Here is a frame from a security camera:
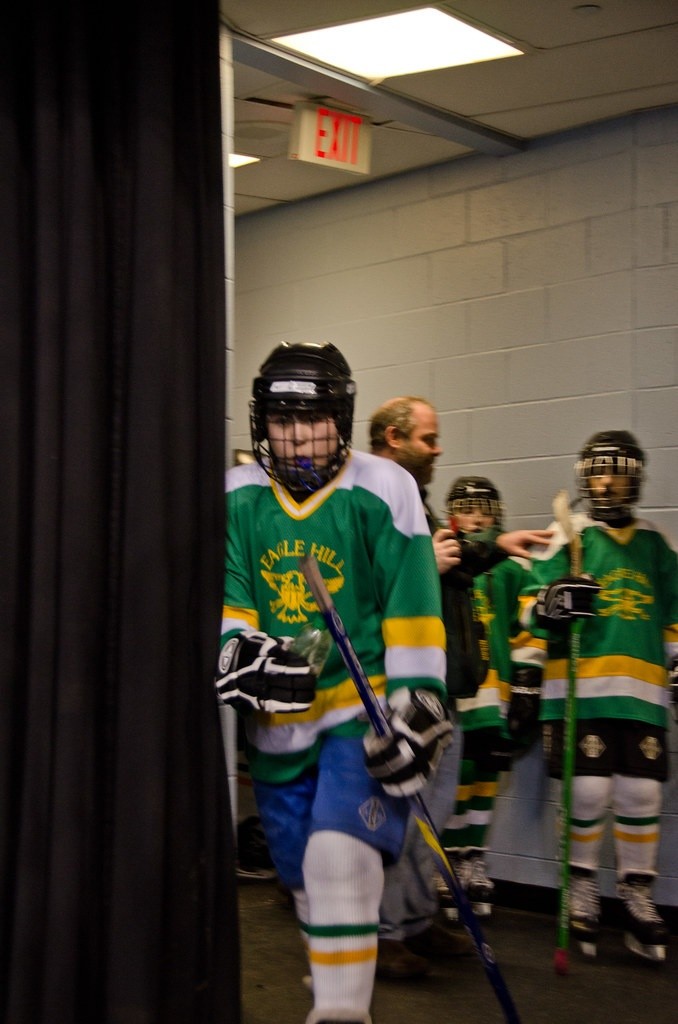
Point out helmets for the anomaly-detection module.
[446,475,504,557]
[248,340,356,490]
[570,430,644,519]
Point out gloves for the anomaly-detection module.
[507,665,543,760]
[361,686,455,798]
[537,577,601,626]
[666,653,678,722]
[213,629,317,714]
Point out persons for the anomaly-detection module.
[519,429,678,965]
[365,395,555,987]
[214,340,453,1024]
[433,473,549,923]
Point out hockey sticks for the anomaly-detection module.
[552,491,581,975]
[297,553,522,1024]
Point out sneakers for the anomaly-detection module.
[434,851,498,908]
[568,867,601,941]
[376,938,429,978]
[403,923,474,958]
[236,814,278,878]
[614,874,669,945]
